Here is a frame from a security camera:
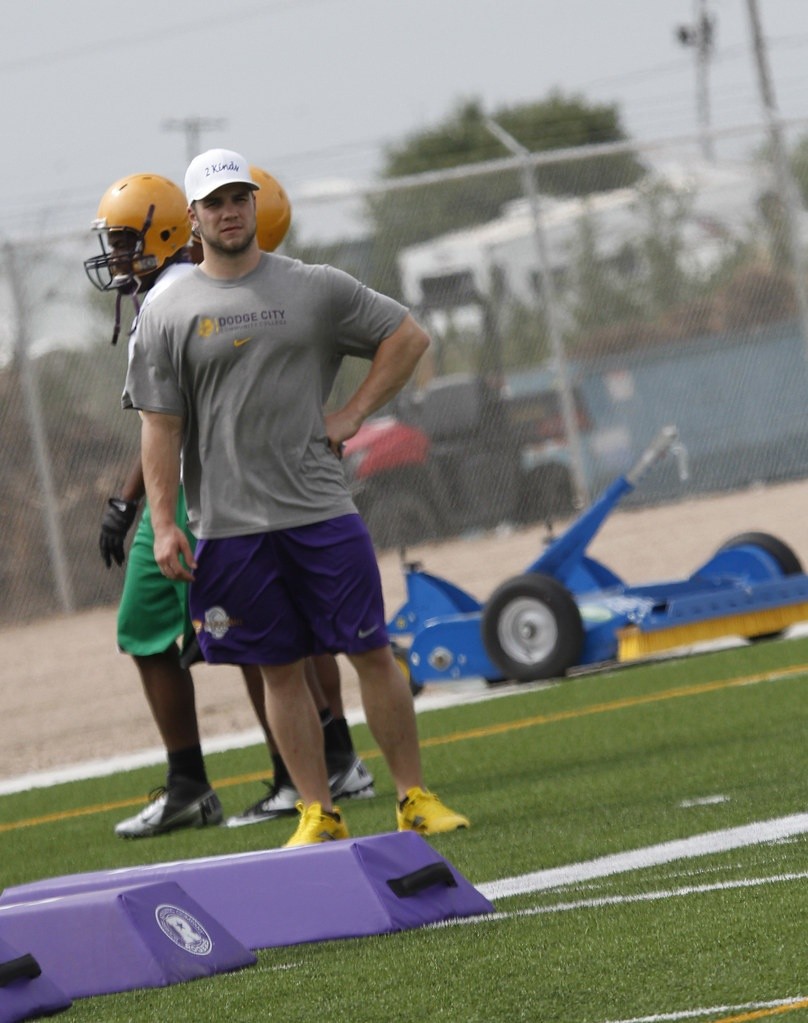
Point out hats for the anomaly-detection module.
[185,149,260,209]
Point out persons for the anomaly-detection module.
[122,148,470,847]
[84,166,377,838]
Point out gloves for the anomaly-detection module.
[98,499,137,569]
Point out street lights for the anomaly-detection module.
[450,101,591,514]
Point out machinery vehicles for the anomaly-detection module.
[341,274,593,552]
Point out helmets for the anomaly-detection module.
[248,166,289,254]
[91,175,192,273]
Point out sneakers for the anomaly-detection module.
[115,774,223,839]
[327,756,376,804]
[395,789,469,833]
[223,782,304,827]
[283,799,349,846]
